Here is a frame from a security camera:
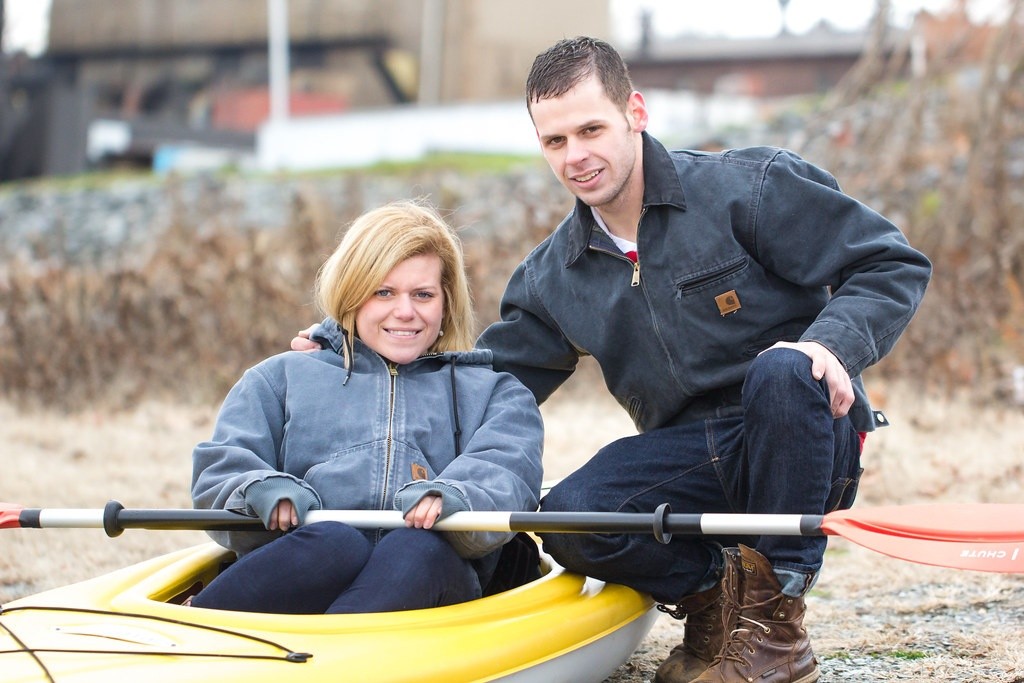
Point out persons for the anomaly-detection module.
[178,198,545,613]
[290,32,932,683]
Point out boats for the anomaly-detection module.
[1,526,667,683]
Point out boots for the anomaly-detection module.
[689,543,822,683]
[650,581,722,683]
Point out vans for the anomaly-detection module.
[0,30,423,187]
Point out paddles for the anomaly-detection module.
[0,503,1023,576]
